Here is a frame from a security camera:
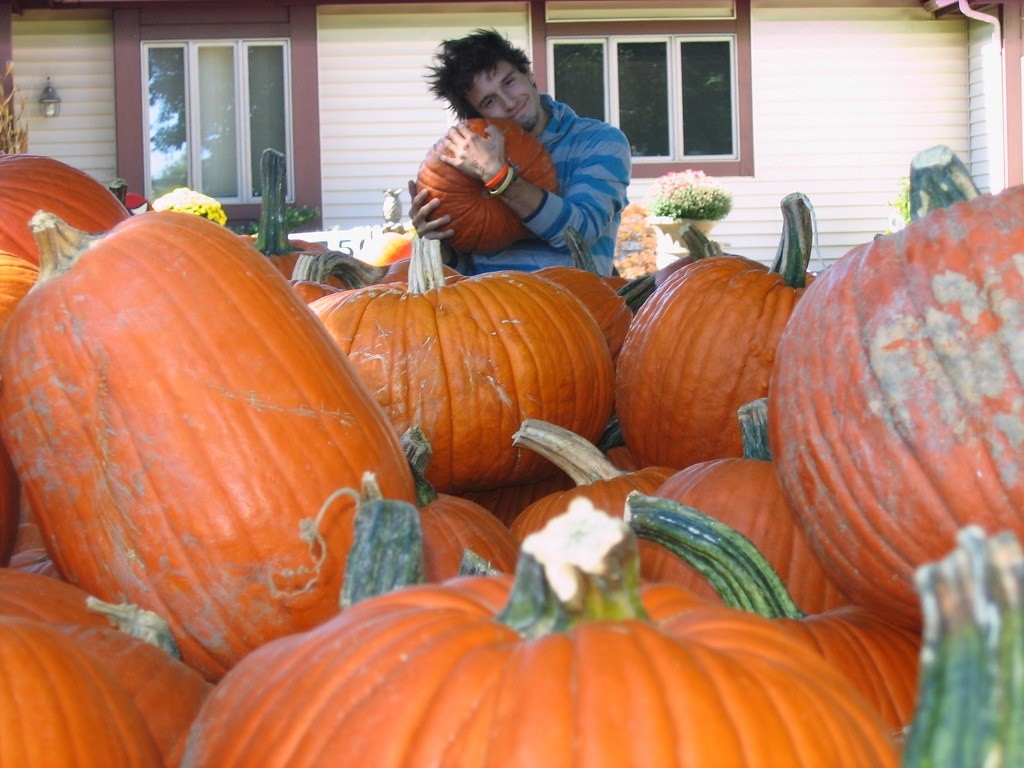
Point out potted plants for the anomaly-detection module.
[653,217,717,261]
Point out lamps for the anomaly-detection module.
[37,74,63,119]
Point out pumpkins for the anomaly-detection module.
[1,120,1024,768]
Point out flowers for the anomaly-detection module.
[151,188,227,226]
[649,168,733,220]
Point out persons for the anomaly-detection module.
[408,27,631,279]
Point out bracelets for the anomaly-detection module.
[485,161,514,197]
